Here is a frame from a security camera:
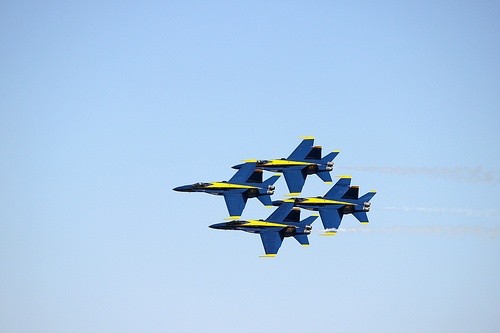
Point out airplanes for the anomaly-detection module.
[171,159,282,220]
[272,175,376,235]
[209,199,319,256]
[230,137,340,196]
